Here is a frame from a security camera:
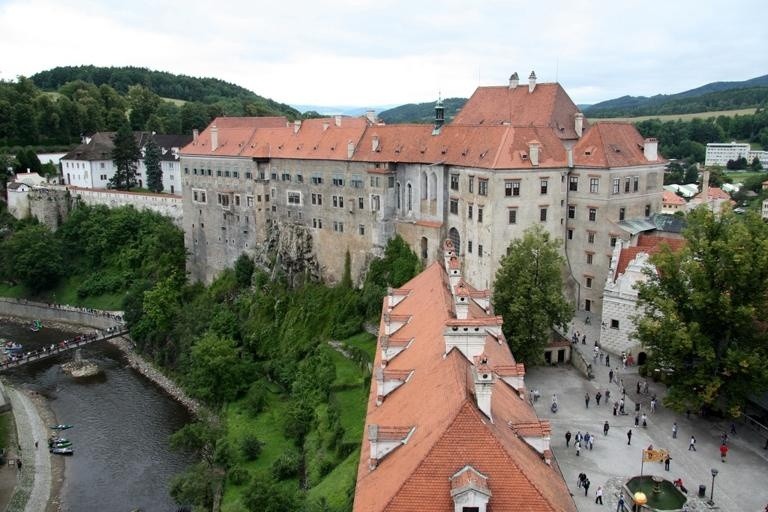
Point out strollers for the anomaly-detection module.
[551,400,558,412]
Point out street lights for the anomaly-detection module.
[654,365,679,407]
[709,468,718,506]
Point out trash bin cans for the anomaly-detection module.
[635,402,640,411]
[595,341,597,346]
[699,485,705,497]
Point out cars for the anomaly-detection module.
[733,208,745,213]
[742,199,751,206]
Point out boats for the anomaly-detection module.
[30,326,40,332]
[0,338,6,347]
[33,319,42,329]
[48,424,73,430]
[5,342,25,350]
[49,435,74,455]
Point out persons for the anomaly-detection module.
[595,485,604,505]
[664,453,672,471]
[672,422,678,439]
[17,458,22,471]
[631,502,641,512]
[570,317,660,444]
[530,386,539,401]
[730,424,736,434]
[647,444,654,458]
[616,491,625,512]
[582,478,590,496]
[551,393,558,407]
[674,477,687,494]
[688,436,696,451]
[5,298,122,361]
[719,431,728,463]
[565,431,595,456]
[578,470,586,488]
[686,409,691,419]
